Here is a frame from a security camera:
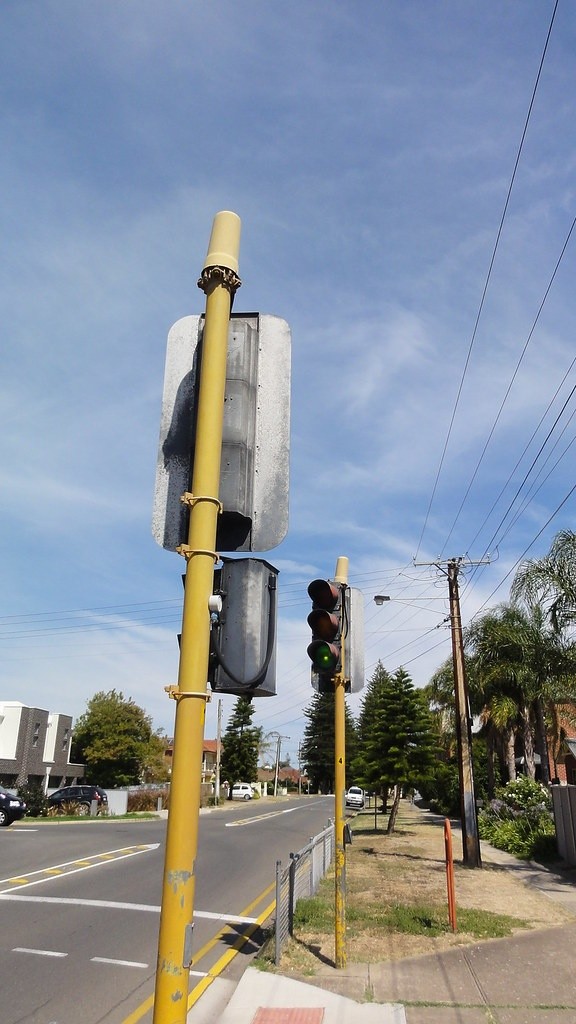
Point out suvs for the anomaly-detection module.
[47,785,110,809]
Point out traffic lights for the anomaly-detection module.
[307,578,351,679]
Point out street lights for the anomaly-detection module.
[372,593,483,873]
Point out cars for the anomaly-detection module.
[226,782,255,800]
[0,785,28,827]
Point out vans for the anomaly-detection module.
[346,786,364,807]
